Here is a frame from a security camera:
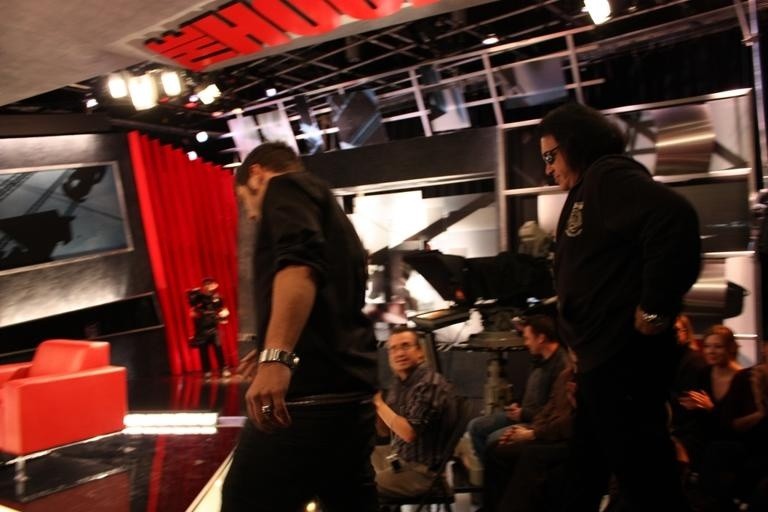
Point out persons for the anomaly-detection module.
[482,341,590,512]
[187,278,231,378]
[374,328,460,512]
[668,315,703,421]
[468,318,578,467]
[220,142,381,512]
[539,104,680,512]
[678,324,758,438]
[721,365,768,512]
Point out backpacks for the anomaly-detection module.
[642,178,702,298]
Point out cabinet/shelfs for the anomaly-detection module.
[493,88,764,370]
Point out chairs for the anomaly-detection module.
[379,416,468,511]
[0,338,128,503]
[436,350,447,378]
[501,346,546,407]
[374,330,438,440]
[448,347,503,427]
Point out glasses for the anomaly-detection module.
[389,343,416,356]
[541,142,563,167]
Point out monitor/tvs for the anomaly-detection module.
[0,159,135,276]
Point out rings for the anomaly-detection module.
[261,404,273,416]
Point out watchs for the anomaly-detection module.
[257,349,302,377]
[642,312,660,325]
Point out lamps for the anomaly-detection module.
[87,99,98,110]
[161,69,184,96]
[128,74,157,111]
[580,0,615,24]
[196,131,210,142]
[196,90,213,106]
[206,83,221,98]
[189,93,197,102]
[265,87,276,95]
[108,72,127,100]
[482,34,498,48]
